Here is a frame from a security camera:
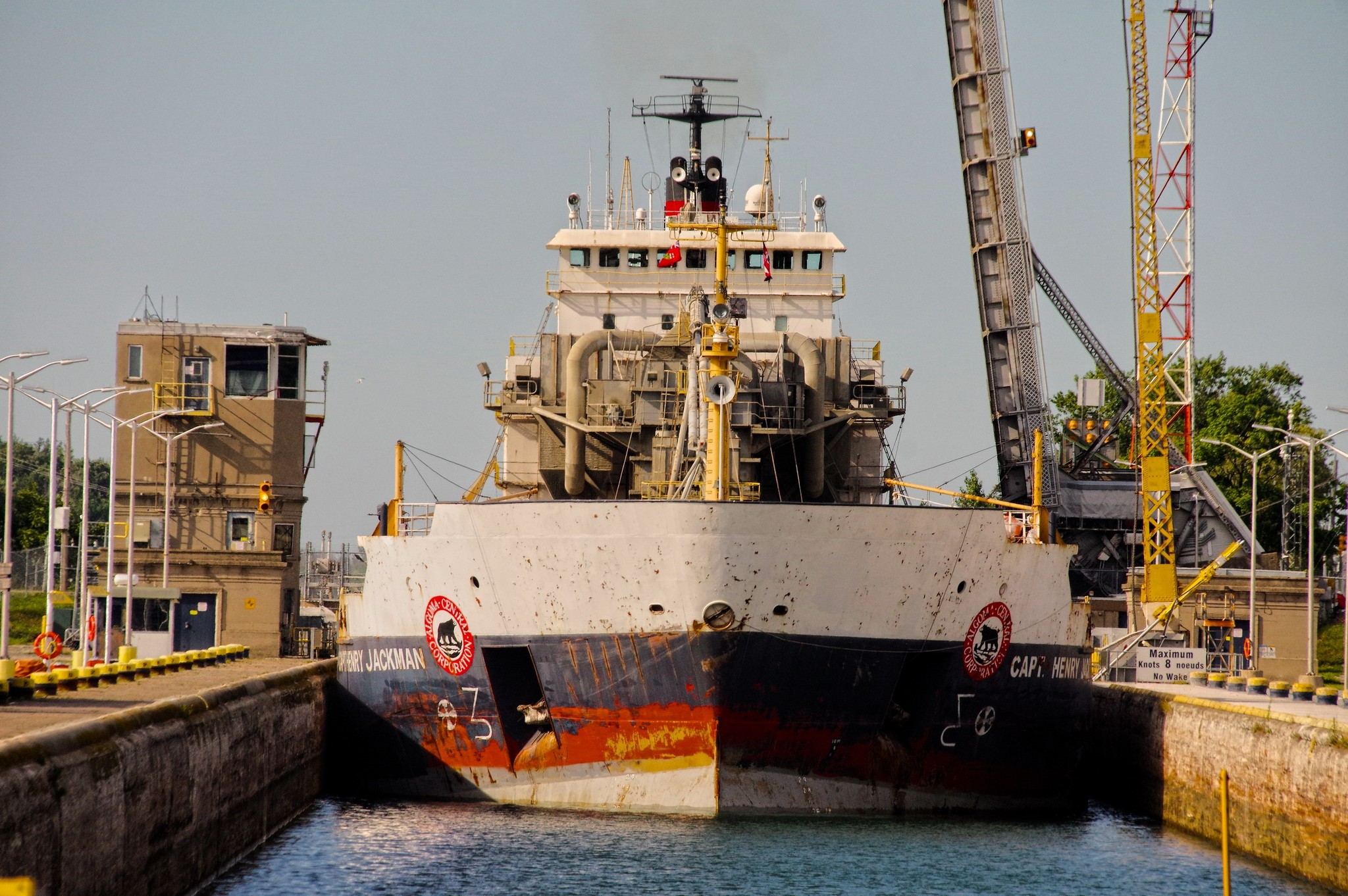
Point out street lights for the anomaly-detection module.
[58,407,178,664]
[0,383,127,673]
[116,420,224,589]
[1199,438,1304,668]
[1251,422,1347,677]
[0,358,90,659]
[21,386,154,666]
[88,408,194,645]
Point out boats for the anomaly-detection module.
[328,76,1095,825]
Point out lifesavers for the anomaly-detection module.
[33,631,63,660]
[1244,638,1251,660]
[88,615,95,641]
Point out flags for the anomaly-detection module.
[762,242,773,282]
[656,239,682,267]
